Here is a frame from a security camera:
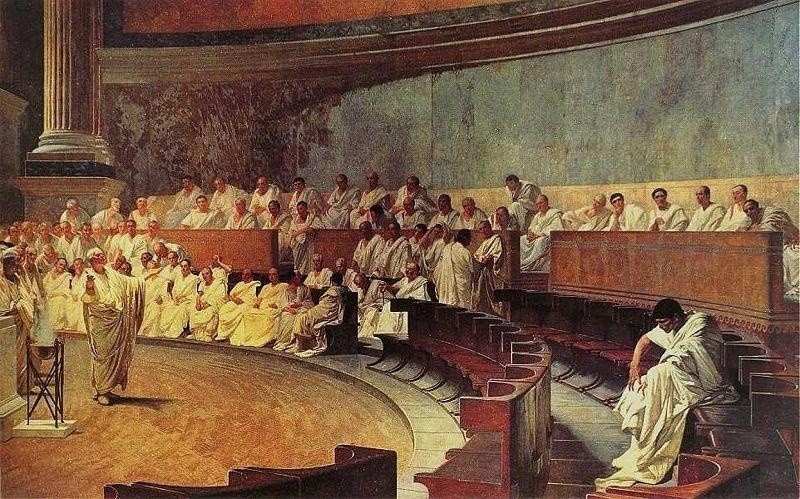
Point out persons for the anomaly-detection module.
[0,247,42,395]
[81,247,148,406]
[1,171,798,373]
[618,297,722,461]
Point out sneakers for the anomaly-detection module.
[97,393,119,405]
